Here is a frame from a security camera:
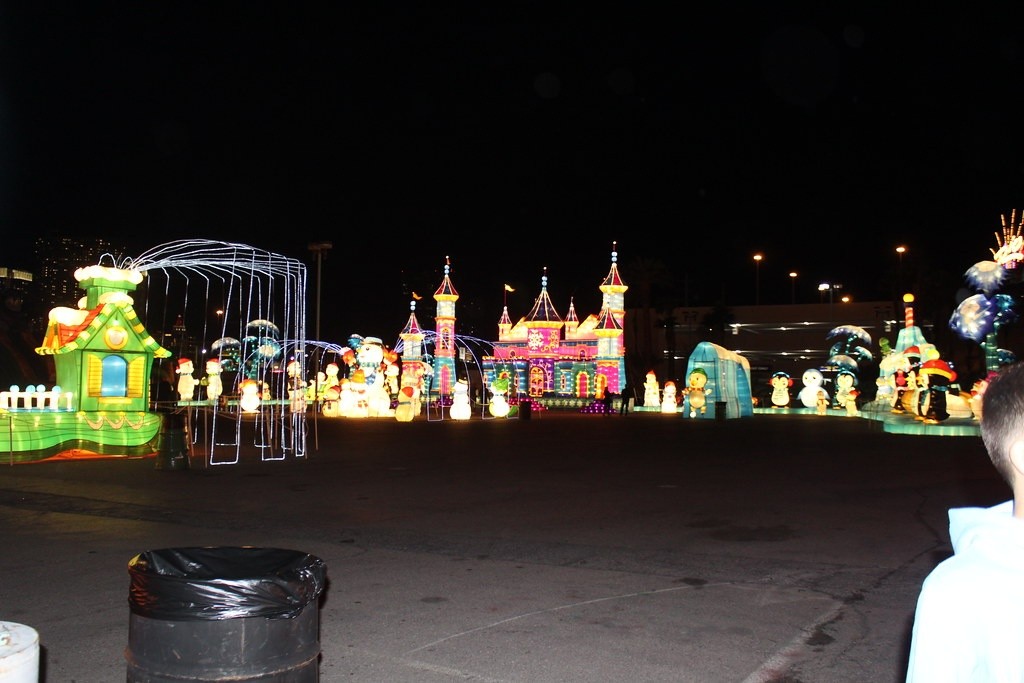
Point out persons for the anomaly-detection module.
[903,363,1024,682]
[618,384,630,416]
[603,387,612,418]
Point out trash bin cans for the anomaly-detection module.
[123,546,330,683]
